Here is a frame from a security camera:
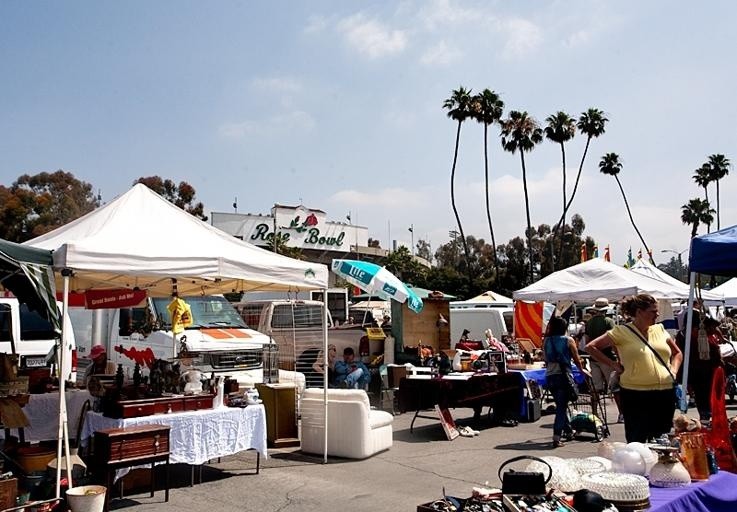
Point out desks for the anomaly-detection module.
[80,404,268,502]
[506,357,592,423]
[646,468,725,511]
[0,389,97,448]
[397,367,528,434]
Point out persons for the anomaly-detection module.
[484,328,509,351]
[312,344,336,388]
[721,309,737,339]
[675,310,725,420]
[380,315,392,331]
[343,316,355,325]
[676,297,700,330]
[583,294,682,443]
[585,297,624,424]
[334,347,372,389]
[725,374,737,401]
[543,316,592,447]
[83,345,117,386]
[677,299,689,337]
[458,330,470,343]
[570,334,579,349]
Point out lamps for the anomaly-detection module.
[581,472,650,509]
[649,447,691,487]
[1,400,33,464]
[436,312,449,349]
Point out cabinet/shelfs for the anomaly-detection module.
[379,382,399,416]
[254,383,301,448]
[93,424,172,512]
[103,391,214,418]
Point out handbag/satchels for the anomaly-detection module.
[547,336,579,401]
[621,324,690,412]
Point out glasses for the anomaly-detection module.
[328,347,336,352]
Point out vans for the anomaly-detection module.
[0,299,77,383]
[450,307,513,348]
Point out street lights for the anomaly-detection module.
[660,250,689,292]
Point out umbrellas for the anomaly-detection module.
[330,260,423,327]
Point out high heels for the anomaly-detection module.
[567,433,578,440]
[553,441,566,447]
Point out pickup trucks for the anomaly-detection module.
[235,300,388,368]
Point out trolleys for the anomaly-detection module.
[560,368,610,442]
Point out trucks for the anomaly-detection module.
[64,294,278,380]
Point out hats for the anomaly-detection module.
[88,345,106,358]
[344,347,356,353]
[586,298,616,311]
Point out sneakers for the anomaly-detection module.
[617,414,624,423]
[459,425,480,435]
[456,427,474,437]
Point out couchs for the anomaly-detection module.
[300,388,394,459]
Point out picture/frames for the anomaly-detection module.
[488,352,504,365]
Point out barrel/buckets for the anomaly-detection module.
[66,484,108,512]
